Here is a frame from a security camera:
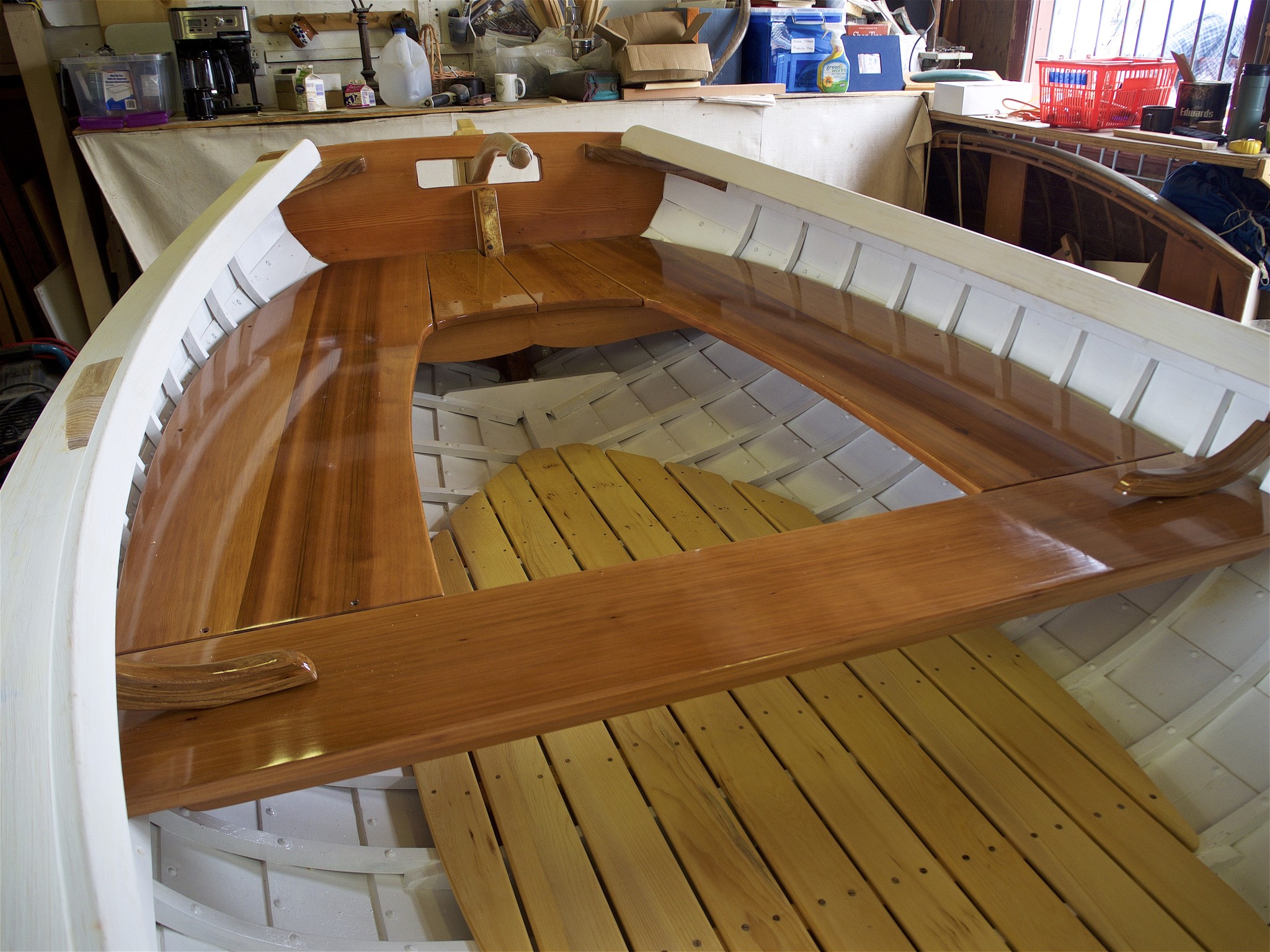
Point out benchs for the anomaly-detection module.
[115,234,1270,812]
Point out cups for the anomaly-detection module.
[445,13,468,44]
[452,77,485,102]
[183,87,218,120]
[1140,105,1177,134]
[495,73,526,102]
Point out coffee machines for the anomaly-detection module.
[168,5,263,121]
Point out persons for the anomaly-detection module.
[1157,10,1250,117]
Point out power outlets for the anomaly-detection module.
[249,43,267,75]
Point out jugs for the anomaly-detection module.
[185,47,239,94]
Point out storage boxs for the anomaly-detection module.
[934,82,1033,116]
[61,52,172,128]
[594,0,845,100]
[274,73,344,111]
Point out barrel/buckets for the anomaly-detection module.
[1174,79,1231,132]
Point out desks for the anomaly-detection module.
[73,88,946,307]
[926,110,1270,310]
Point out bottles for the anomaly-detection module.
[1227,63,1270,142]
[378,28,432,107]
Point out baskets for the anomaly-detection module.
[1035,56,1179,132]
